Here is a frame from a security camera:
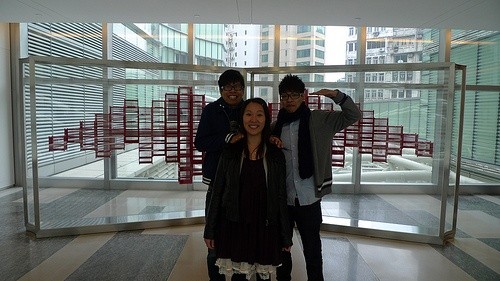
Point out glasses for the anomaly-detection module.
[280,91,303,101]
[221,83,243,91]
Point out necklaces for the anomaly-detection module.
[248,144,258,154]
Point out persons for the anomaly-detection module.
[204,98,293,281]
[270,74,361,281]
[194,70,284,281]
